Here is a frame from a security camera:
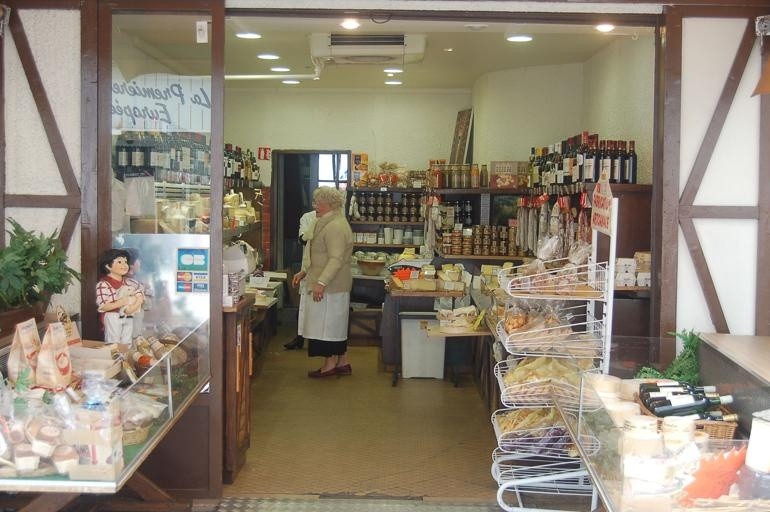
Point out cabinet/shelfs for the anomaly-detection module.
[339,178,655,423]
[214,287,257,486]
[483,190,621,512]
[115,166,278,365]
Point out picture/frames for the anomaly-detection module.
[446,105,476,167]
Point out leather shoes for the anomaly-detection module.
[335,363,352,375]
[308,367,336,377]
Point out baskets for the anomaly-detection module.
[632,392,739,452]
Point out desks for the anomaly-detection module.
[0,303,217,512]
[543,332,769,512]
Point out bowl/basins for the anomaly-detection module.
[351,225,424,246]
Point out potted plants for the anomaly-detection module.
[0,211,90,340]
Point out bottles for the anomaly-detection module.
[508,226,517,242]
[440,200,473,227]
[526,130,639,186]
[112,127,261,188]
[356,191,425,222]
[122,331,184,367]
[427,159,490,189]
[518,245,524,256]
[637,374,743,428]
[508,242,517,257]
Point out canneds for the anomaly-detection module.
[359,192,425,222]
[442,200,517,256]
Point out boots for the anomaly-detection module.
[283,336,304,349]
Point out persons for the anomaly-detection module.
[96,248,143,349]
[282,210,320,350]
[293,186,353,377]
